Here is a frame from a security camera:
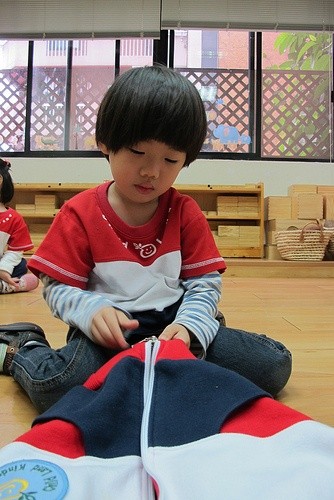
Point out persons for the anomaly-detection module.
[0,64,293,415]
[0,158,39,294]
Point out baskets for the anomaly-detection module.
[316,219,334,252]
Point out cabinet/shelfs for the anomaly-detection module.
[11,182,266,258]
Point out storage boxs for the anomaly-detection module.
[16,195,69,256]
[203,195,259,217]
[265,184,334,259]
[211,225,260,247]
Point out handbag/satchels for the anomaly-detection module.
[275,222,333,261]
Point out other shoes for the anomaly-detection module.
[215,312,226,326]
[0,321,46,376]
